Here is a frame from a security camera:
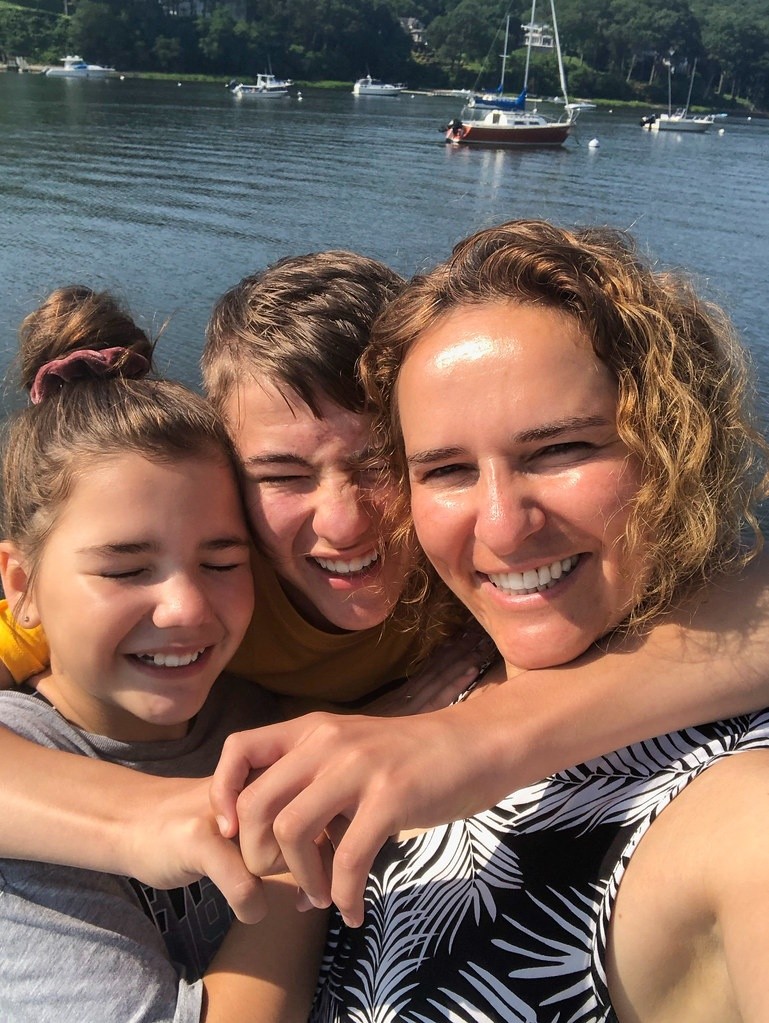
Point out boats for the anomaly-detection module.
[564,103,600,111]
[224,55,295,98]
[351,75,407,96]
[40,54,116,78]
[639,47,714,132]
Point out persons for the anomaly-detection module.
[0,282,491,1023]
[305,217,768,1023]
[1,246,769,930]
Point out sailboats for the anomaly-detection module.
[439,0,576,147]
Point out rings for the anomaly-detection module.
[403,694,412,702]
